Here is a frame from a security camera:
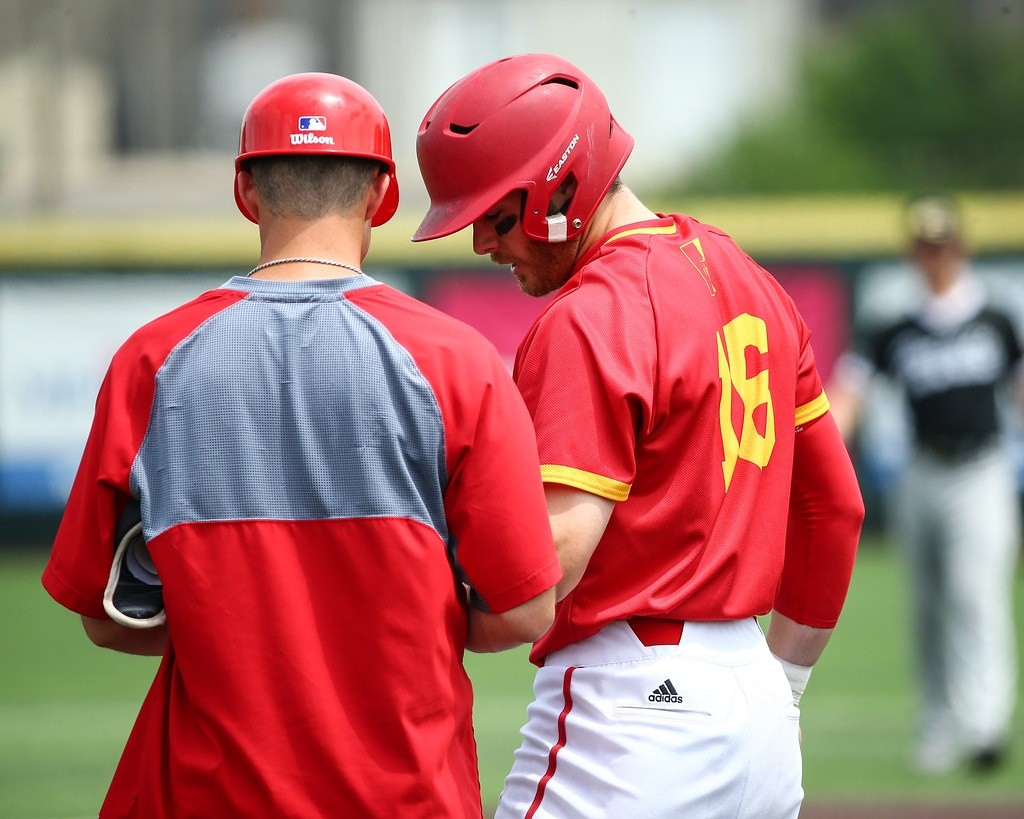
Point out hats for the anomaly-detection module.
[902,193,964,245]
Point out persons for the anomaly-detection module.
[40,73,564,819]
[408,53,865,819]
[818,190,1024,780]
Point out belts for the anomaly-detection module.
[625,615,684,646]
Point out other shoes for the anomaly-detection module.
[969,742,1012,775]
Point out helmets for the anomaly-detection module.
[234,73,400,228]
[410,53,635,242]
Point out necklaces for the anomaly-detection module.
[246,258,363,277]
